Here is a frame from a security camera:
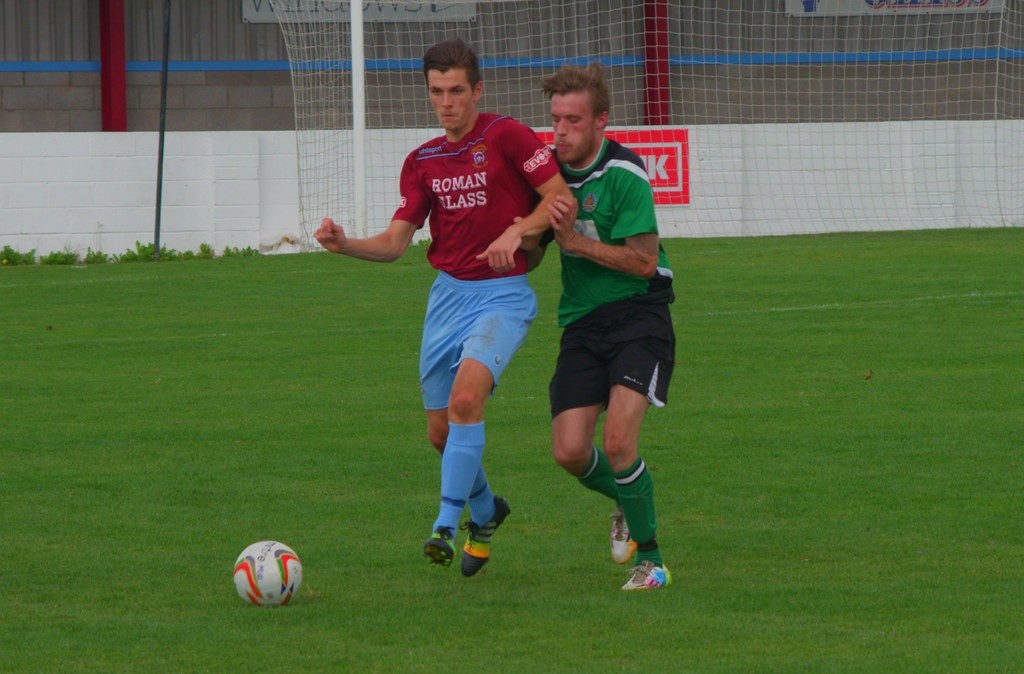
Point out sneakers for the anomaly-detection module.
[608,506,637,564]
[620,560,672,590]
[459,497,511,577]
[423,525,457,567]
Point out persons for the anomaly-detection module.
[315,38,571,578]
[512,66,677,592]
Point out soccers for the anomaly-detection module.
[231,539,303,604]
